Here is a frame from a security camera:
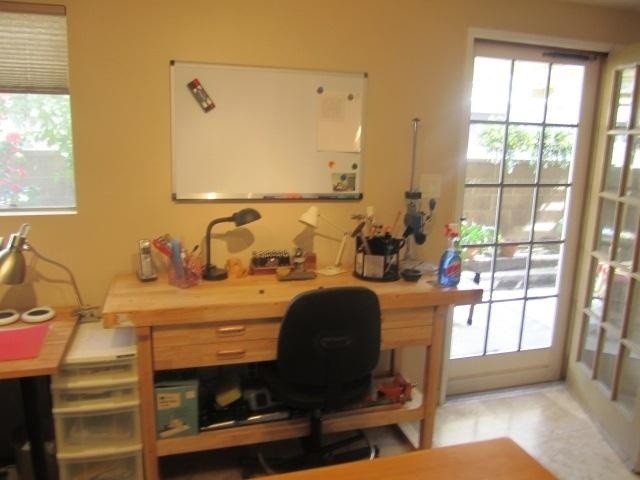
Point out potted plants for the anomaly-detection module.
[460,221,487,261]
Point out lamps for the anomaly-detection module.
[0,234,95,321]
[299,206,350,276]
[203,209,262,280]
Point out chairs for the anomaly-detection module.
[256,288,380,476]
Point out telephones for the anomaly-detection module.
[138,240,157,280]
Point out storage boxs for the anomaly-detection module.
[156,376,199,439]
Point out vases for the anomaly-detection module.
[500,240,519,256]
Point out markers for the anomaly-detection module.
[263,193,363,201]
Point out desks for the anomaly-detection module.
[254,438,561,480]
[0,306,81,479]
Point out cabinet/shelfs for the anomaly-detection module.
[51,309,144,480]
[104,271,482,480]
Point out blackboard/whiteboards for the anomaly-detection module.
[169,60,368,201]
